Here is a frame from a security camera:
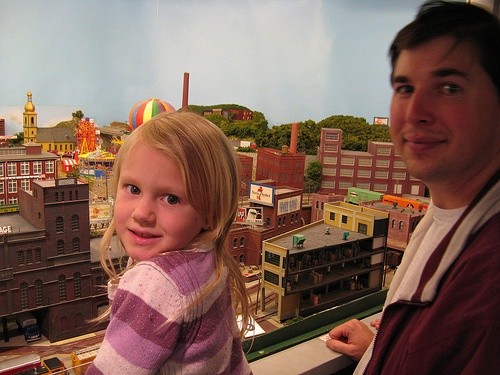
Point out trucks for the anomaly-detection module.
[0,355,66,375]
[71,342,103,375]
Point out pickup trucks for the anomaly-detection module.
[17,315,41,342]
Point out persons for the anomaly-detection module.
[85,111,253,375]
[326,0,500,375]
